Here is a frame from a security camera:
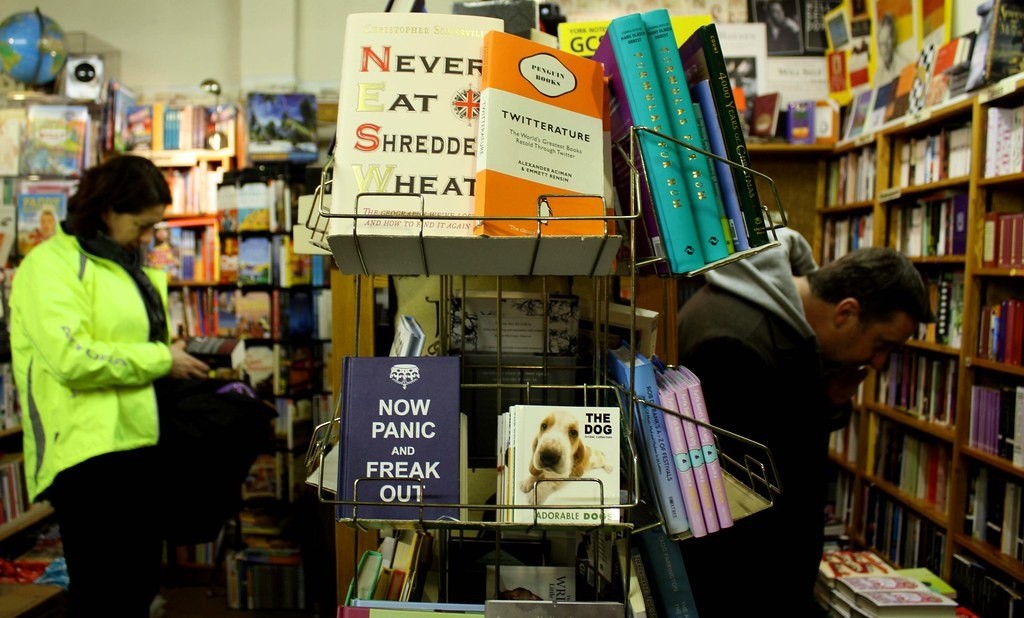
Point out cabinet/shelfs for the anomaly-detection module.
[1,92,336,617]
[812,72,1023,618]
[305,118,788,618]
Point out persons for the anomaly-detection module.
[652,226,940,618]
[8,154,215,618]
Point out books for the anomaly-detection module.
[326,13,772,618]
[689,0,1024,617]
[0,88,329,616]
[169,333,247,373]
